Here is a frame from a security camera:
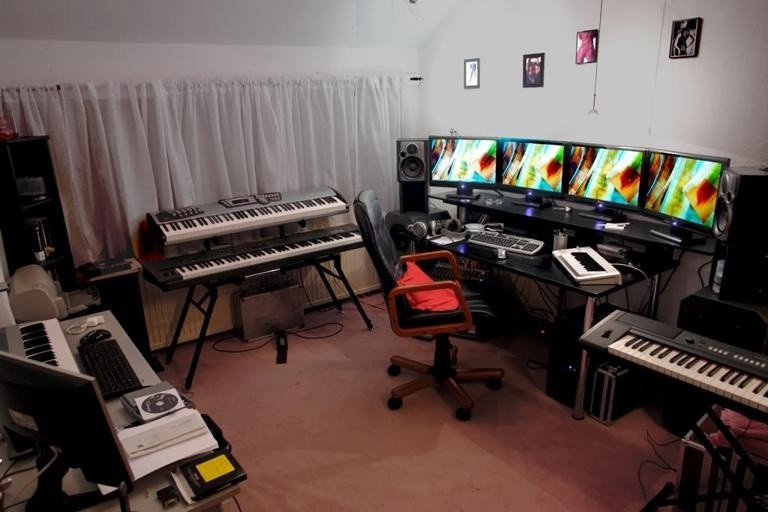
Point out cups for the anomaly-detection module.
[553,235,568,252]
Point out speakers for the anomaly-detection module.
[709,166,768,300]
[396,138,429,215]
[384,211,428,257]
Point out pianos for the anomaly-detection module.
[145,185,349,245]
[579,309,768,422]
[141,221,365,293]
[1,318,82,459]
[552,246,623,286]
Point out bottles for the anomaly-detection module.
[33,227,47,264]
[712,260,725,294]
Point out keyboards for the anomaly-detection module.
[467,228,544,256]
[75,339,143,402]
[429,266,492,282]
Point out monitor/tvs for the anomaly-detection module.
[566,141,647,222]
[0,348,135,512]
[500,137,567,208]
[642,147,730,244]
[428,137,503,198]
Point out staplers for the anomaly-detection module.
[484,223,504,232]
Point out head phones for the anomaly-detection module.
[408,220,443,238]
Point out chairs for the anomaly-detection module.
[353,189,505,421]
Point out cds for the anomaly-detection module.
[141,393,178,413]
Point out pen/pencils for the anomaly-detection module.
[553,228,567,237]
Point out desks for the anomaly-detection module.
[399,194,724,420]
[77,255,152,361]
[0,309,241,512]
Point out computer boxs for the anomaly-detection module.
[545,304,625,410]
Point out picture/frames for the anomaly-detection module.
[523,53,545,88]
[464,58,480,89]
[669,16,703,59]
[575,29,599,64]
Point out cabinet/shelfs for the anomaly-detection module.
[0,135,81,295]
[167,252,373,389]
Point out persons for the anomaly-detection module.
[469,63,476,81]
[673,26,695,56]
[527,58,539,83]
[576,30,595,63]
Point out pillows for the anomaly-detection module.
[397,262,461,312]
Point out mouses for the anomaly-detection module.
[76,328,111,343]
[495,248,508,260]
